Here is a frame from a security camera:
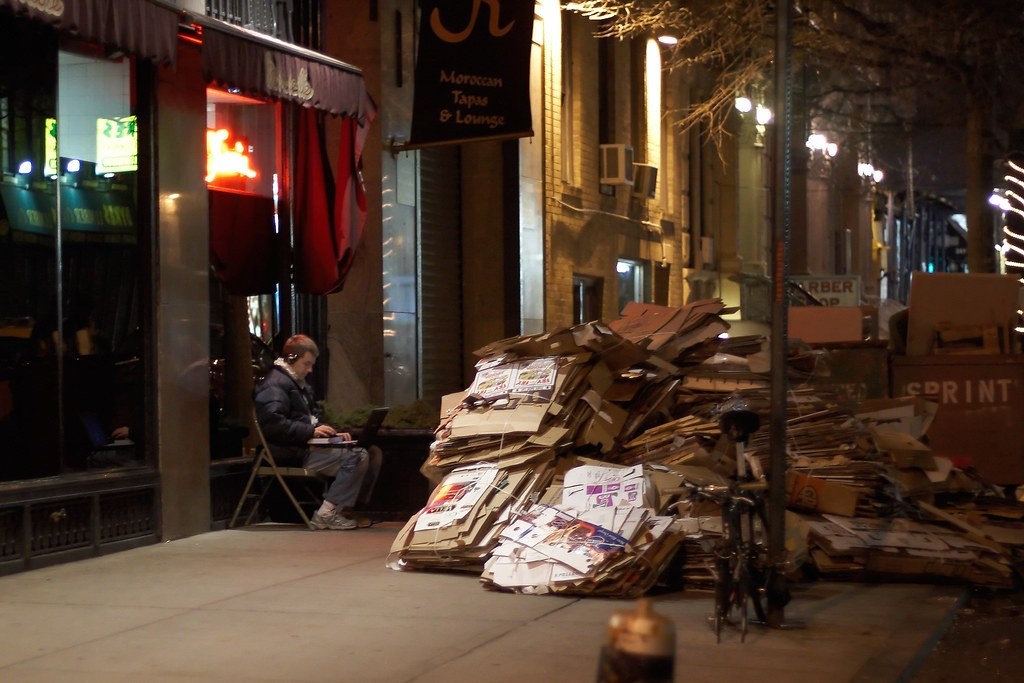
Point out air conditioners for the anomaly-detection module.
[632,162,658,200]
[597,144,634,186]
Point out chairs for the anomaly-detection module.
[229,408,330,532]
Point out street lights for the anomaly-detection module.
[20,157,83,471]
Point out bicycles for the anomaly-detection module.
[659,397,790,644]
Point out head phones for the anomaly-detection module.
[287,353,297,364]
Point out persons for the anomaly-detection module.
[86,421,144,470]
[252,335,382,529]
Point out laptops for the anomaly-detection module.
[307,406,391,448]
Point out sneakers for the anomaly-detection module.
[309,508,357,529]
[345,512,374,527]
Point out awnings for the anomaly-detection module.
[0,0,367,128]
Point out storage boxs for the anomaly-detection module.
[389,270,1024,599]
